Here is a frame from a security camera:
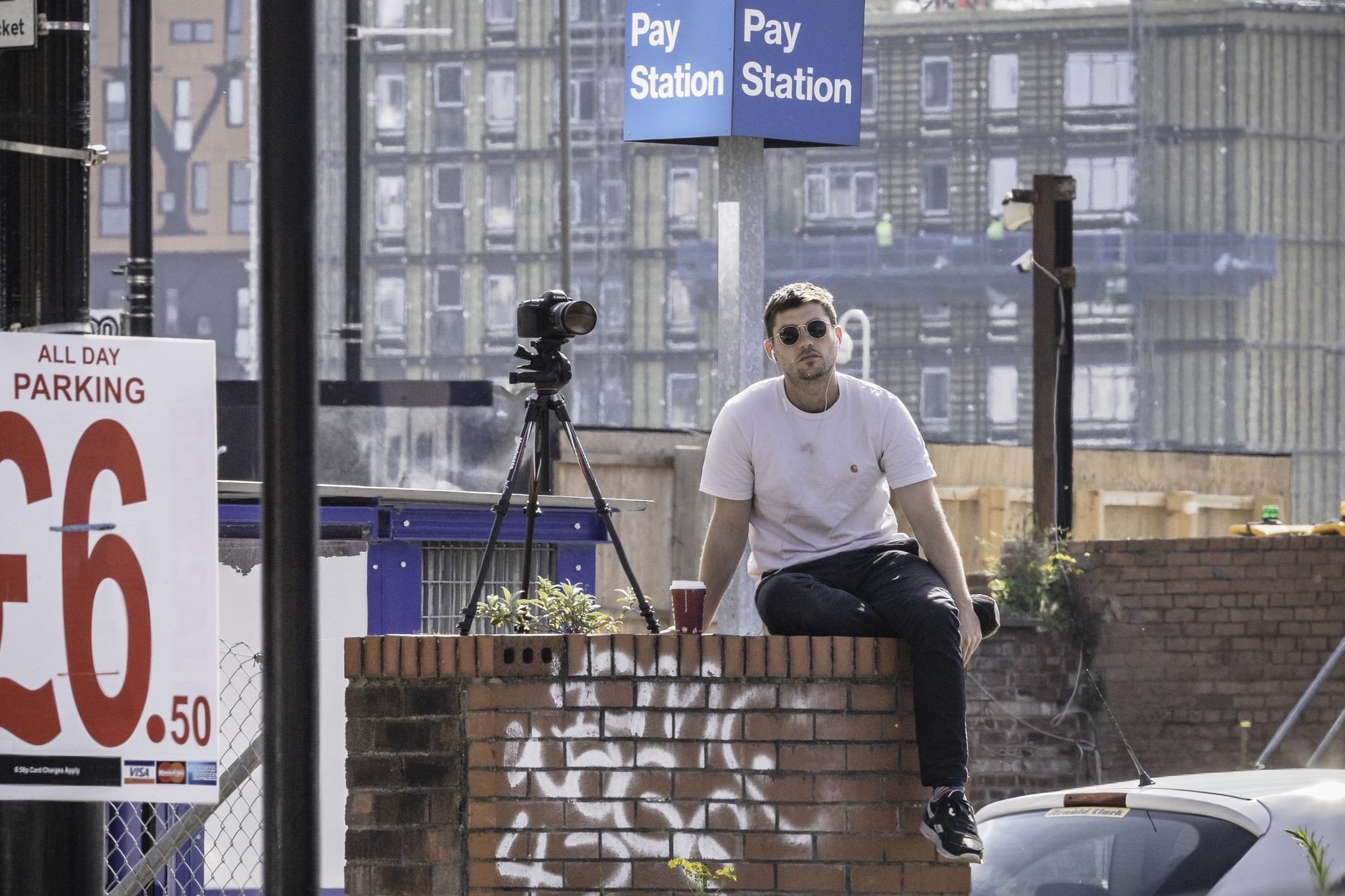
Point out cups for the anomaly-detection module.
[670,580,707,634]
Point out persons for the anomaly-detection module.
[659,281,1002,864]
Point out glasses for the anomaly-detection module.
[769,319,835,346]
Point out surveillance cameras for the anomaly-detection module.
[1011,247,1034,273]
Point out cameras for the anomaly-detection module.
[517,288,598,339]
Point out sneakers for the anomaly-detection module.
[971,593,1001,640]
[918,789,985,864]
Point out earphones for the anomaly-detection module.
[771,348,776,362]
[836,336,840,349]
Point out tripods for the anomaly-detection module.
[454,336,660,637]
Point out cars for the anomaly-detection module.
[968,767,1345,895]
